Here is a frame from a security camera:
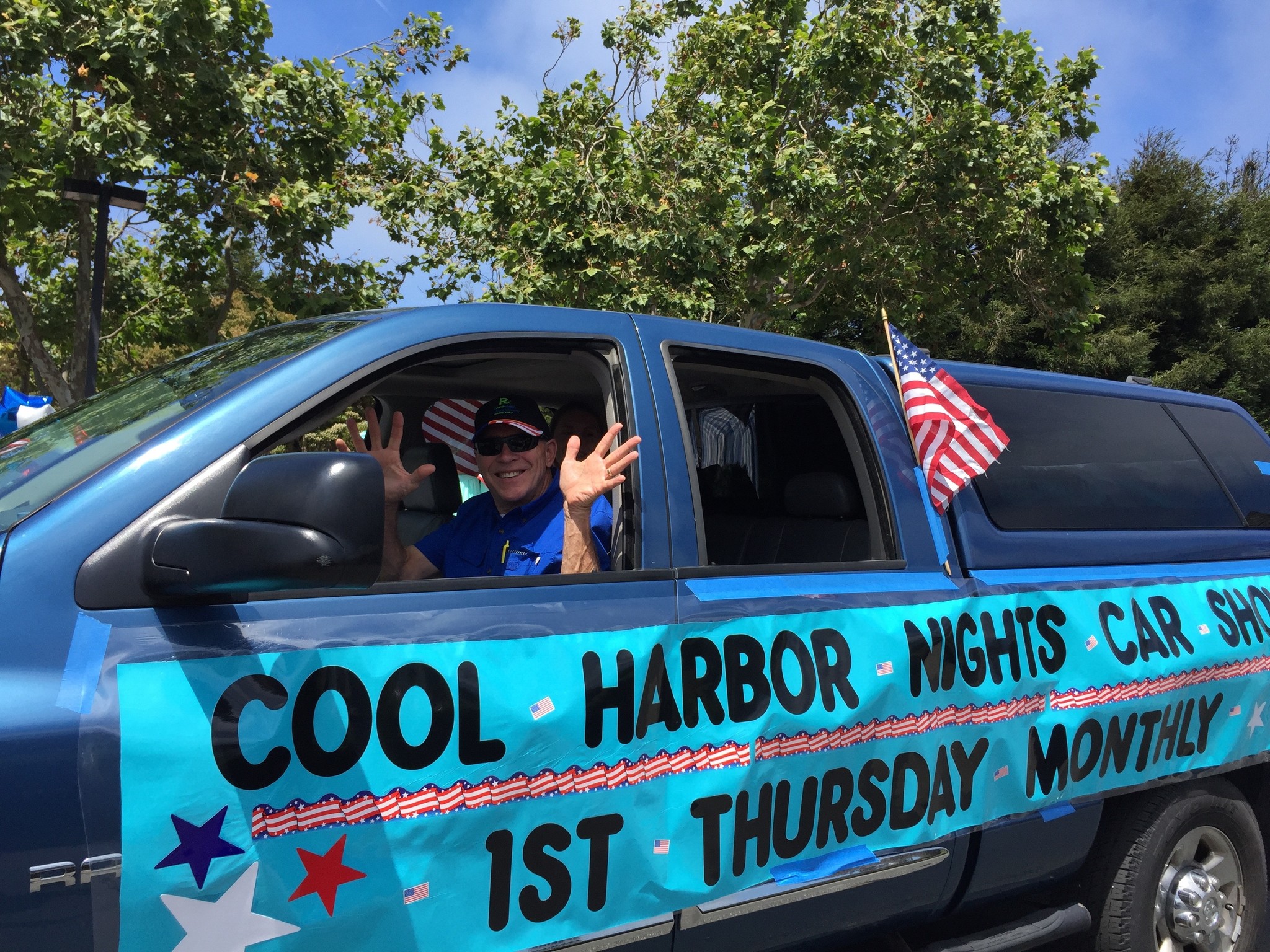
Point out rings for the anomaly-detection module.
[607,466,615,477]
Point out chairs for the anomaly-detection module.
[397,443,462,581]
[697,464,872,566]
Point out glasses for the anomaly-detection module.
[475,433,548,456]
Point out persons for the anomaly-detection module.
[329,395,644,581]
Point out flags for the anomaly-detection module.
[422,395,488,478]
[885,318,1011,514]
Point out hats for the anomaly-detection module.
[471,396,553,443]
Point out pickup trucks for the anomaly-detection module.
[1,296,1270,952]
